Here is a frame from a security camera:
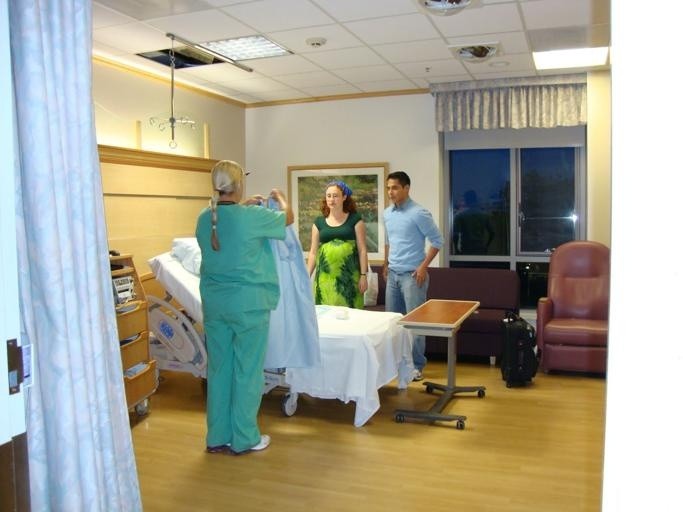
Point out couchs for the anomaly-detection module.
[362,263,522,368]
[536,240,610,379]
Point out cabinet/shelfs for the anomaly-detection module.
[105,252,161,420]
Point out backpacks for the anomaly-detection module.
[501,313,541,389]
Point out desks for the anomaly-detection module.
[393,299,486,433]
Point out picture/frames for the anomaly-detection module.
[285,161,390,267]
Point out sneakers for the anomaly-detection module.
[413,369,424,380]
[228,434,271,456]
[206,443,231,453]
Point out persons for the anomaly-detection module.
[382,171,444,381]
[195,160,294,456]
[306,181,368,309]
[453,190,495,255]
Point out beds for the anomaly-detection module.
[139,236,405,419]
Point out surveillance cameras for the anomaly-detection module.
[305,37,326,48]
